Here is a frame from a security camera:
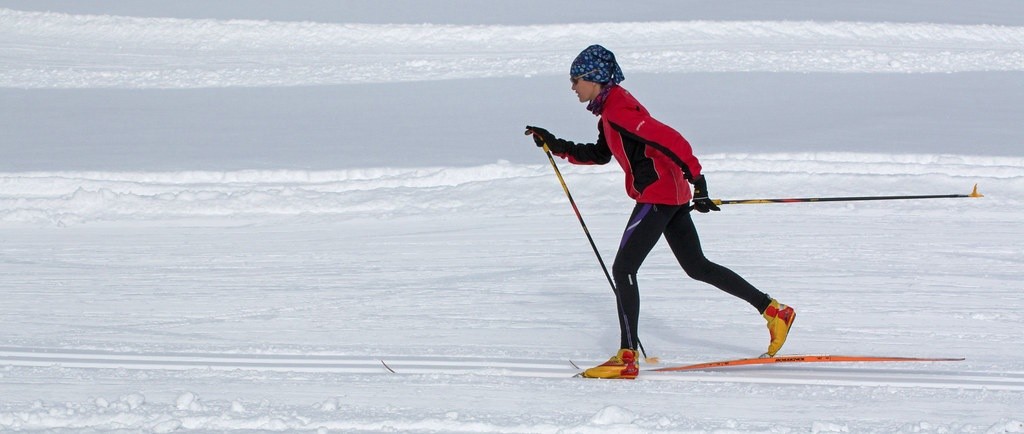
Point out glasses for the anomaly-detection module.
[570,69,602,85]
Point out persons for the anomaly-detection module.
[524,46,793,383]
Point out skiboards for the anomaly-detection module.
[375,353,968,386]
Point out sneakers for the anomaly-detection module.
[762,298,796,357]
[580,348,640,379]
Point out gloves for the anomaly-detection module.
[691,175,721,214]
[524,125,557,151]
